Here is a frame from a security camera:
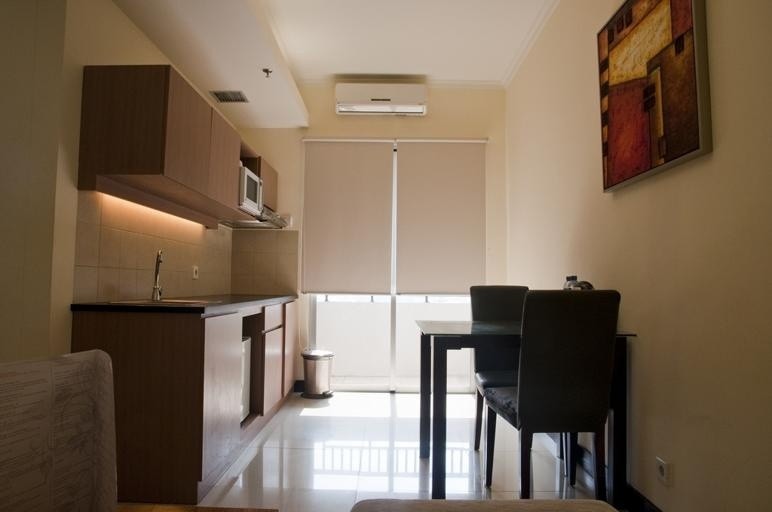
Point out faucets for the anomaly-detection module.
[151,247,164,302]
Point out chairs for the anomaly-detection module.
[468,284,527,452]
[484,286,623,502]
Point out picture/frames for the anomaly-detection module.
[596,1,716,194]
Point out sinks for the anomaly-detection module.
[108,298,210,305]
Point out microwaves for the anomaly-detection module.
[240,166,264,218]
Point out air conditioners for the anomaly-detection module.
[337,76,426,117]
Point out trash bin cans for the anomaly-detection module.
[300,346,336,400]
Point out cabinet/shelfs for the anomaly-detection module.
[243,299,303,419]
[242,154,279,226]
[77,63,239,226]
[71,309,243,504]
